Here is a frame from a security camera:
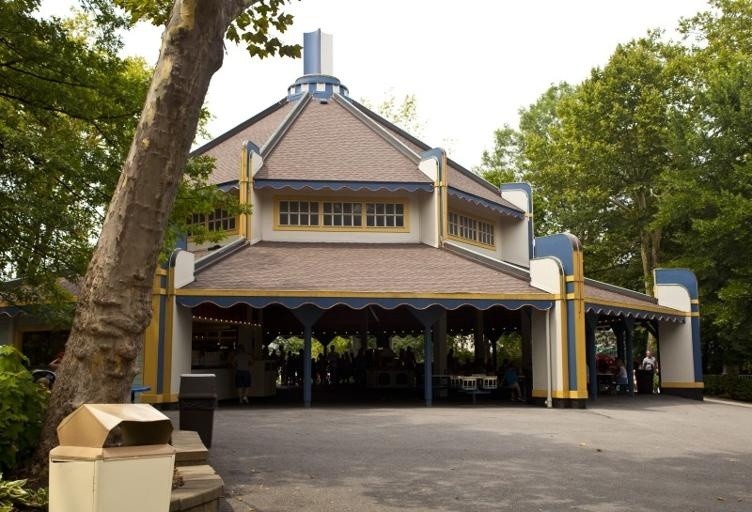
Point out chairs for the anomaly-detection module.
[449,373,498,405]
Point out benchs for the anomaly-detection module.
[420,385,450,400]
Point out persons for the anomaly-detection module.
[193,334,532,404]
[587,350,660,399]
[23,332,69,388]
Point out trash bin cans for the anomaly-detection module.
[49,404,177,512]
[635,369,654,393]
[178,373,217,449]
